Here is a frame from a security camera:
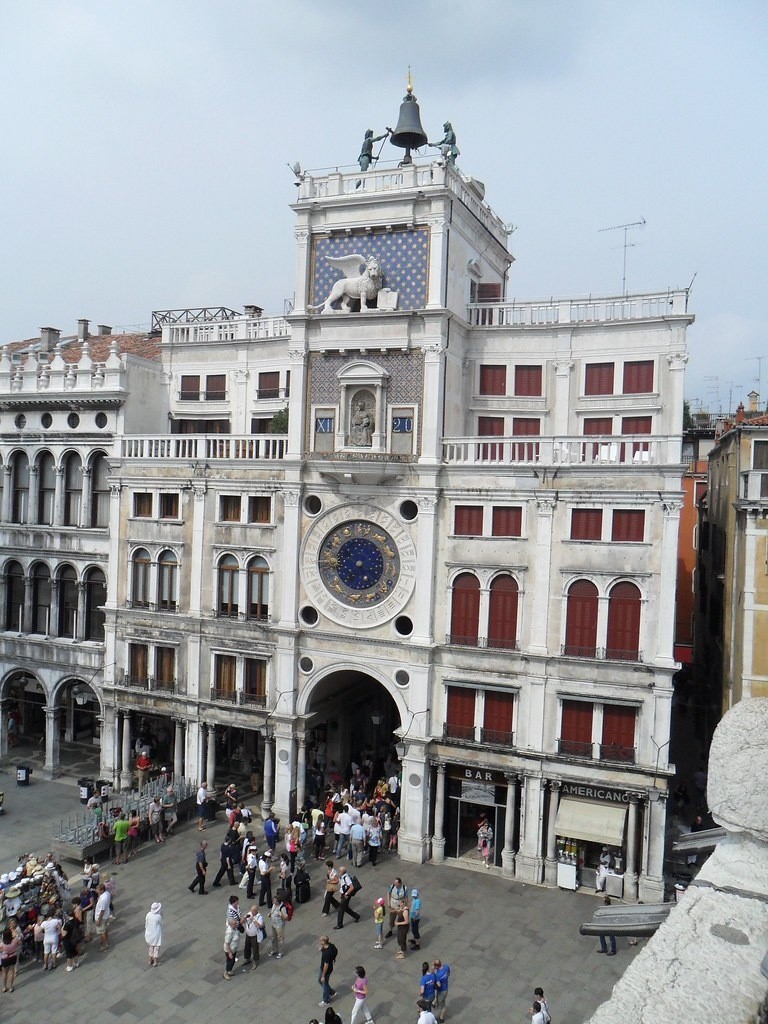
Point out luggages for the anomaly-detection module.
[295,879,311,903]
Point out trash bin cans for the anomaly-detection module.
[77,777,95,805]
[16,766,33,787]
[95,780,113,804]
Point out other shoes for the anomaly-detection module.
[374,942,384,949]
[3,987,13,992]
[199,891,208,895]
[32,934,110,971]
[408,938,420,950]
[188,886,195,892]
[247,893,256,899]
[222,950,287,981]
[149,957,163,967]
[310,844,349,860]
[397,951,405,959]
[213,883,221,887]
[230,881,238,885]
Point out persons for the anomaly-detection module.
[249,753,262,794]
[419,959,450,1024]
[529,987,551,1024]
[428,122,460,164]
[687,816,703,867]
[318,936,338,1006]
[630,901,644,945]
[188,782,293,907]
[351,402,373,446]
[309,1007,342,1024]
[596,847,610,894]
[373,878,421,959]
[223,896,288,980]
[416,999,437,1024]
[87,790,140,864]
[597,896,617,956]
[149,787,177,842]
[286,745,403,872]
[321,861,361,930]
[0,852,115,993]
[144,902,163,967]
[356,129,390,189]
[477,809,493,869]
[39,727,46,752]
[8,709,21,749]
[351,966,374,1024]
[131,729,170,795]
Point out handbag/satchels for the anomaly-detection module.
[280,902,293,921]
[257,928,267,944]
[326,883,338,893]
[301,813,309,829]
[342,876,362,896]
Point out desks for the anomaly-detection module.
[52,773,199,863]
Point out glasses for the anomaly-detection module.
[46,856,50,857]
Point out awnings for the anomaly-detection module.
[554,796,630,846]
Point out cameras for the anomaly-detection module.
[246,915,250,918]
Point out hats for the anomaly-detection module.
[264,851,272,856]
[411,889,418,898]
[151,902,161,913]
[0,854,56,898]
[249,846,257,850]
[374,897,385,905]
